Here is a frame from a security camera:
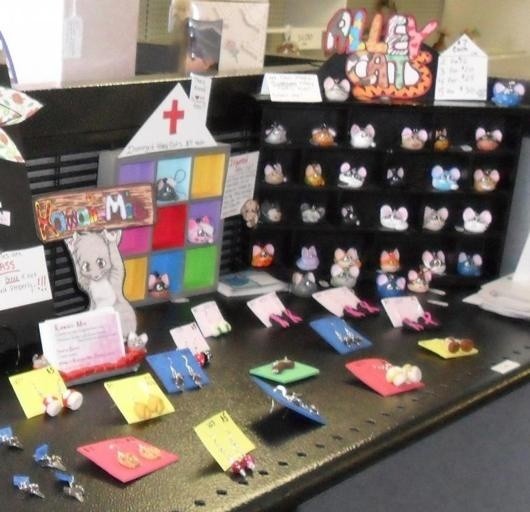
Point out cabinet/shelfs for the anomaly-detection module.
[234,100,527,286]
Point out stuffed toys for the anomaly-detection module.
[490,80,526,107]
[263,163,498,192]
[265,123,288,144]
[309,123,503,151]
[299,203,492,234]
[240,198,282,229]
[251,245,275,268]
[292,246,483,298]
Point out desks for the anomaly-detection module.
[3,281,530,511]
